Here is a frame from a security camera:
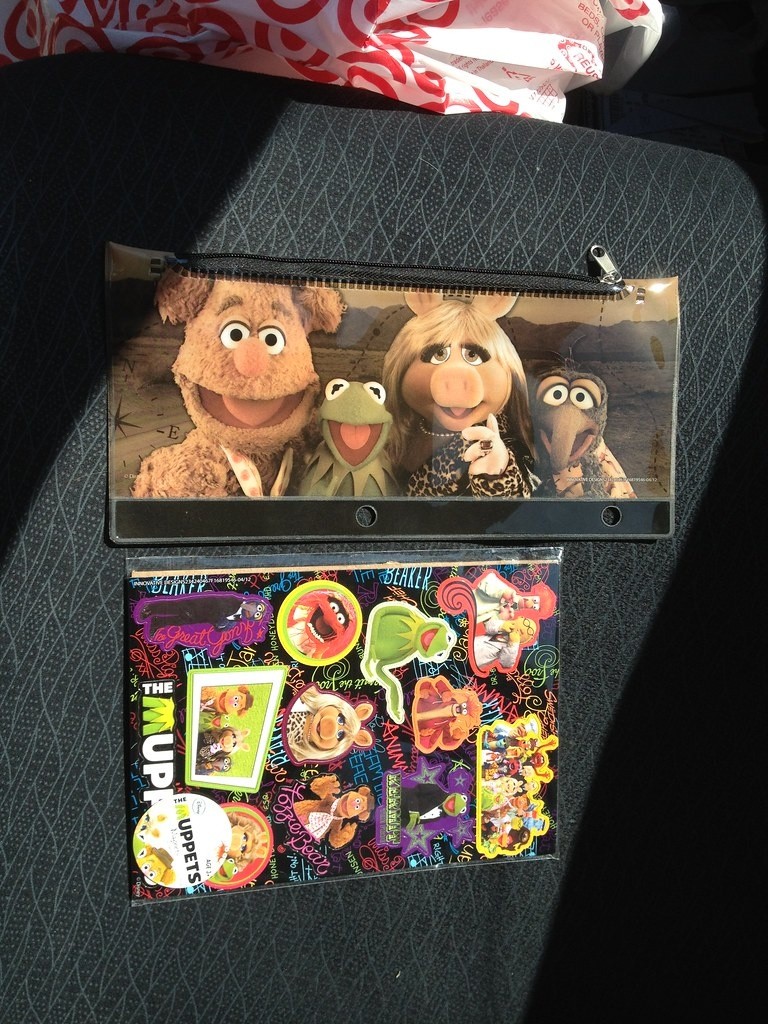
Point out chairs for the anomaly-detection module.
[0,52,767,1024]
